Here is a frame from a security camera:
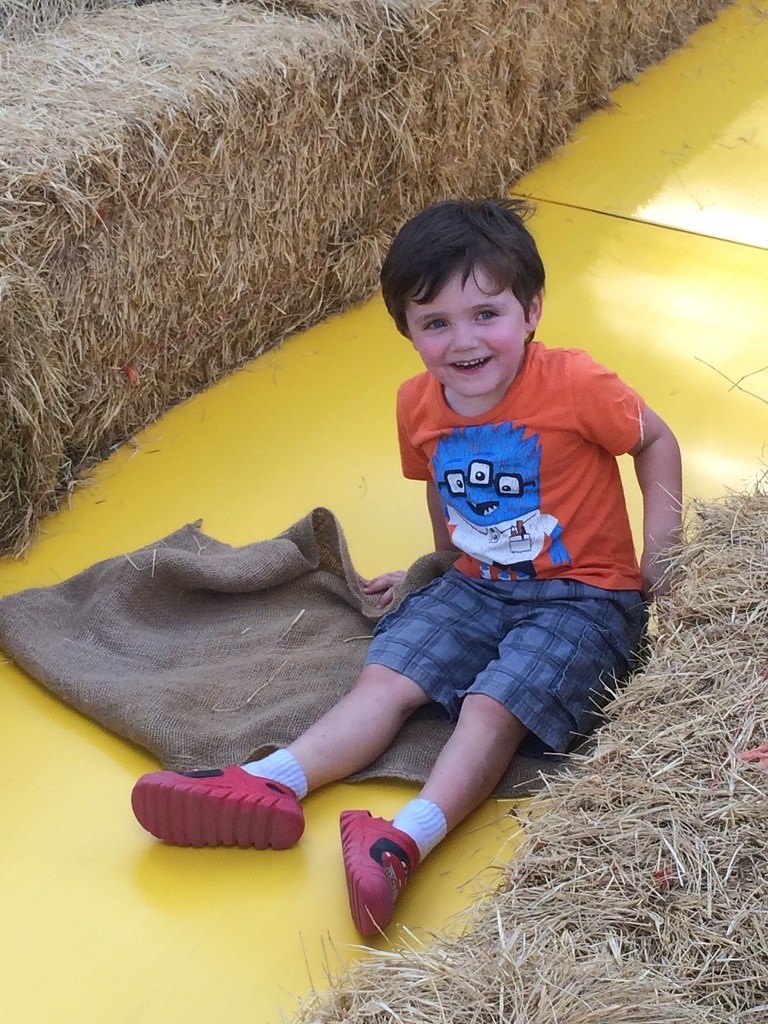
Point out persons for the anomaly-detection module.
[130,194,682,937]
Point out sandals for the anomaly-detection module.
[130,766,304,851]
[340,810,421,937]
